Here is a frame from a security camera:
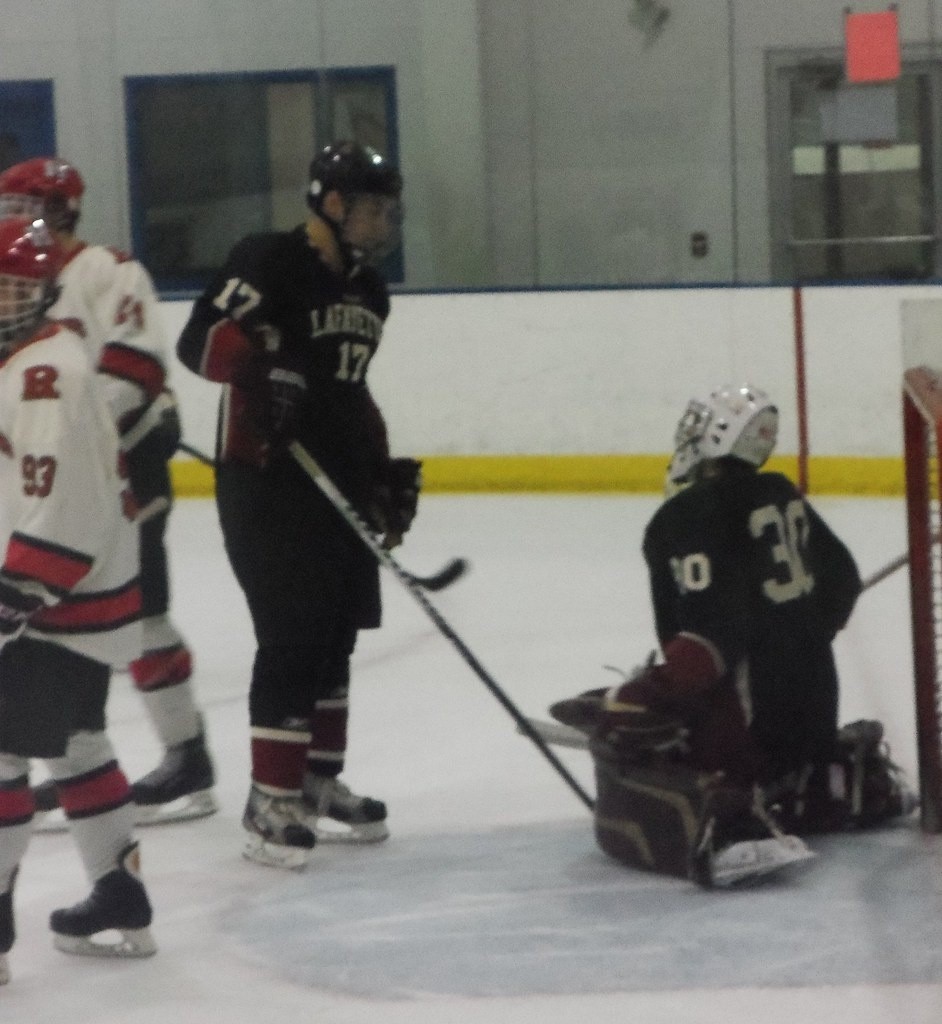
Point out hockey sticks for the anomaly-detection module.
[287,438,596,812]
[176,439,465,594]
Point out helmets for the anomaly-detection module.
[673,382,780,465]
[0,216,57,279]
[1,158,86,204]
[311,141,406,195]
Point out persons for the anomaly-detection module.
[0,153,225,828]
[548,379,923,894]
[0,189,162,987]
[175,134,403,873]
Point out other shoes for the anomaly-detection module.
[128,743,217,828]
[240,791,313,869]
[34,783,67,829]
[0,894,14,985]
[49,870,155,957]
[304,776,390,844]
